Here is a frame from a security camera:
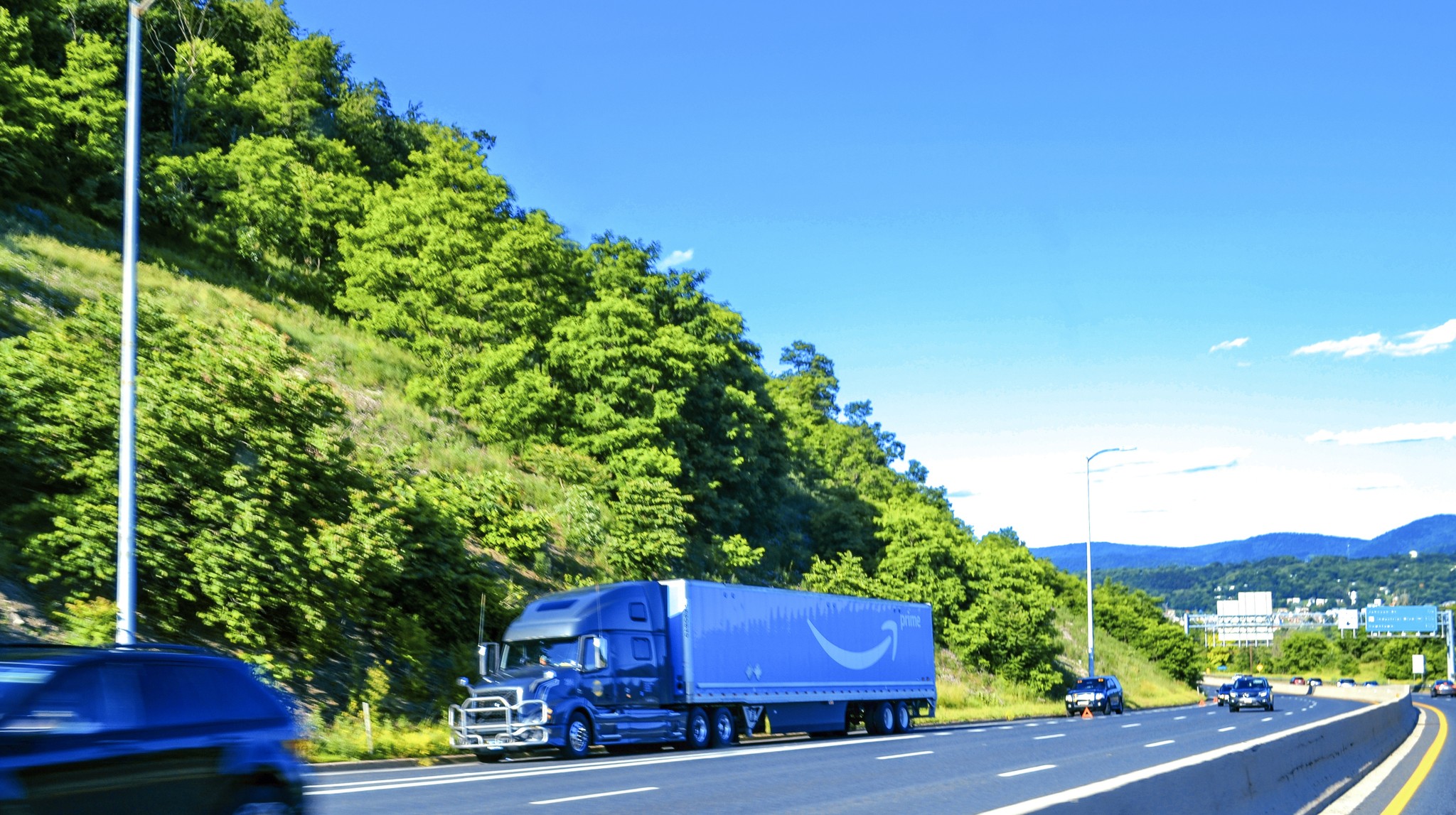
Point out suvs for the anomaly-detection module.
[1064,674,1124,718]
[1227,677,1273,713]
[1336,679,1357,687]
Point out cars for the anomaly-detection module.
[0,640,313,815]
[1362,681,1379,687]
[1289,677,1305,685]
[1216,683,1234,706]
[1306,678,1322,686]
[1429,679,1456,698]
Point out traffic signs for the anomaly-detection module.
[1338,609,1358,629]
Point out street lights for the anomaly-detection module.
[1085,447,1137,677]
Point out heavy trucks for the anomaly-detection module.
[445,581,939,762]
